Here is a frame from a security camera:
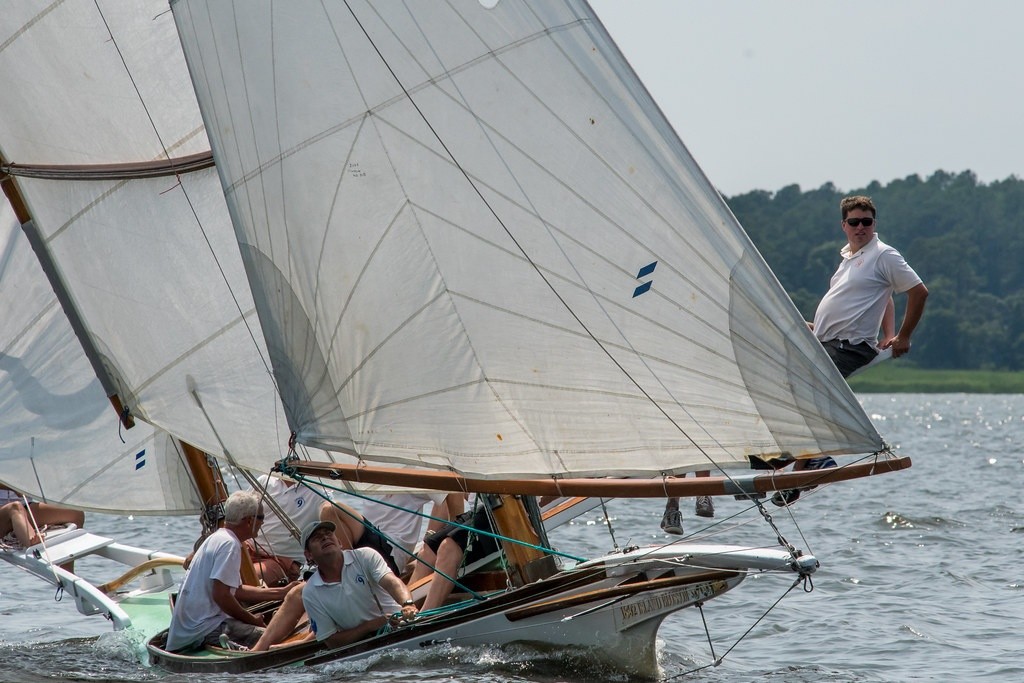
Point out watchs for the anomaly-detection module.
[402,598,415,607]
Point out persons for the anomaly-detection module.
[770,196,929,507]
[659,469,714,536]
[163,488,301,655]
[250,492,466,653]
[299,520,421,649]
[0,483,85,575]
[406,504,502,612]
[244,475,327,584]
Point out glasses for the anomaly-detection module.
[844,217,876,227]
[242,514,264,520]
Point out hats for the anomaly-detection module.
[301,521,337,551]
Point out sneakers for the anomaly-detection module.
[694,496,715,517]
[660,511,684,535]
[771,488,800,507]
[802,456,838,470]
[218,633,250,652]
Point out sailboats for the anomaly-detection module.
[0,0,915,682]
